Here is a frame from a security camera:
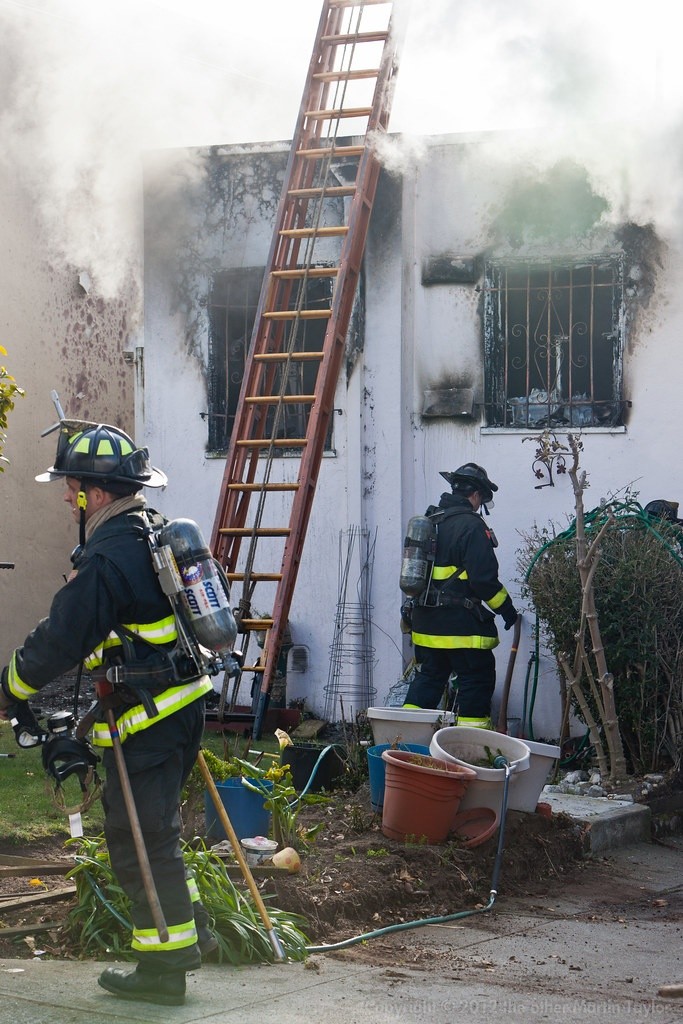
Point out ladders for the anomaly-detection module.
[207,0,407,745]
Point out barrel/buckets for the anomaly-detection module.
[367,743,430,815]
[429,726,561,828]
[205,777,273,842]
[367,707,455,745]
[381,750,477,845]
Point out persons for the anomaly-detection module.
[399,463,518,730]
[0,419,219,1006]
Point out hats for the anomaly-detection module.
[439,463,498,509]
[35,423,168,489]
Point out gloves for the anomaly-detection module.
[0,665,28,720]
[502,605,518,630]
[7,702,49,749]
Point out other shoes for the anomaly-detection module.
[197,925,219,956]
[98,960,186,1006]
